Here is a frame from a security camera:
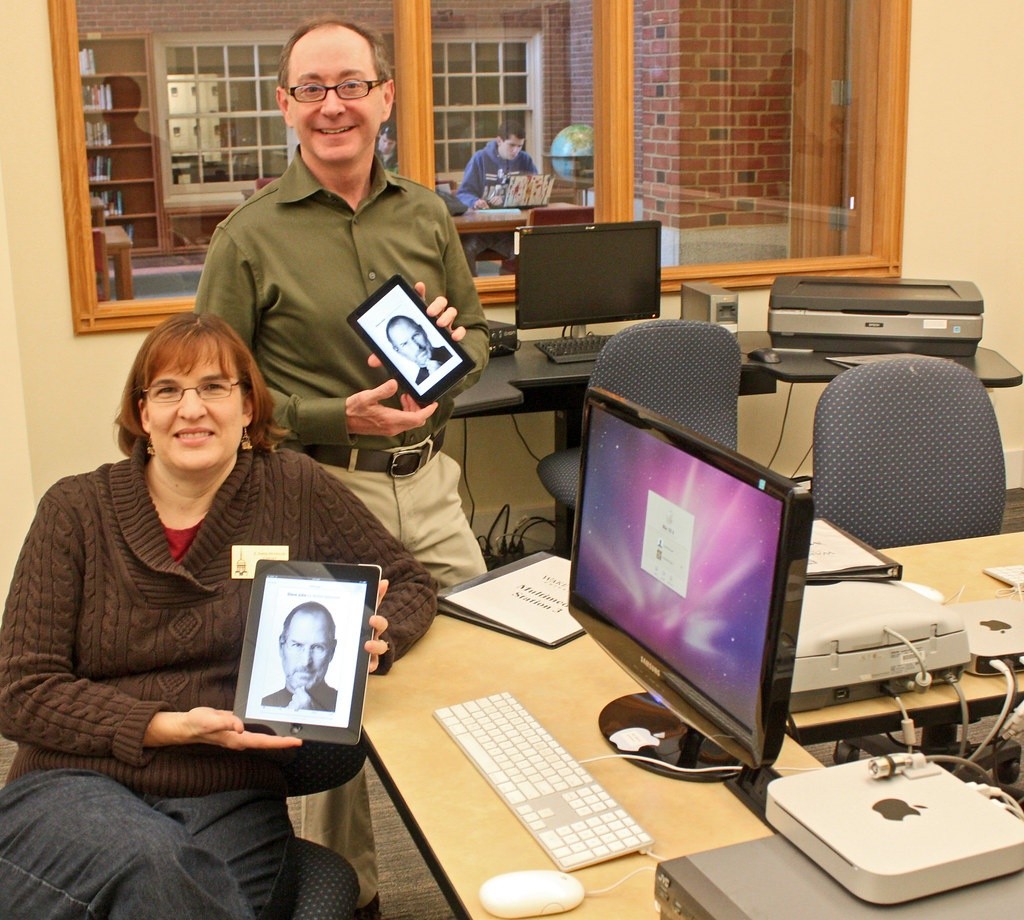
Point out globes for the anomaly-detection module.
[542,122,594,187]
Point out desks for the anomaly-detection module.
[450,346,1019,548]
[93,225,133,299]
[90,197,108,227]
[361,532,1024,920]
[453,202,580,233]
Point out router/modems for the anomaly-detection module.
[765,751,1024,904]
[943,598,1024,676]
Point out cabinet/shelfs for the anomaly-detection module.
[77,31,165,258]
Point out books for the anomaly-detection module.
[77,48,135,244]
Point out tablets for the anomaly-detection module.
[232,559,382,744]
[346,274,476,410]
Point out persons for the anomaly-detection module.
[386,316,453,385]
[454,119,539,276]
[261,601,338,712]
[377,124,397,173]
[0,312,441,920]
[196,18,488,920]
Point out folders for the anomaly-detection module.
[434,551,585,650]
[805,518,903,581]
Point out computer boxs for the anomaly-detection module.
[680,283,740,337]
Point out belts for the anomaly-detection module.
[273,424,446,478]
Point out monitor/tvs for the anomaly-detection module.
[569,387,813,788]
[516,219,661,330]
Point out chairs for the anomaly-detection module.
[92,230,111,301]
[500,206,595,274]
[814,351,1007,550]
[536,322,739,557]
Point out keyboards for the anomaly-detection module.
[534,334,615,363]
[432,692,657,872]
[984,565,1024,592]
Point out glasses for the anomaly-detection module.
[284,78,385,103]
[142,379,241,403]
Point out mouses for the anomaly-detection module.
[480,869,586,919]
[747,347,781,364]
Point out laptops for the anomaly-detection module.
[489,175,556,209]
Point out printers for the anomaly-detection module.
[766,274,984,357]
